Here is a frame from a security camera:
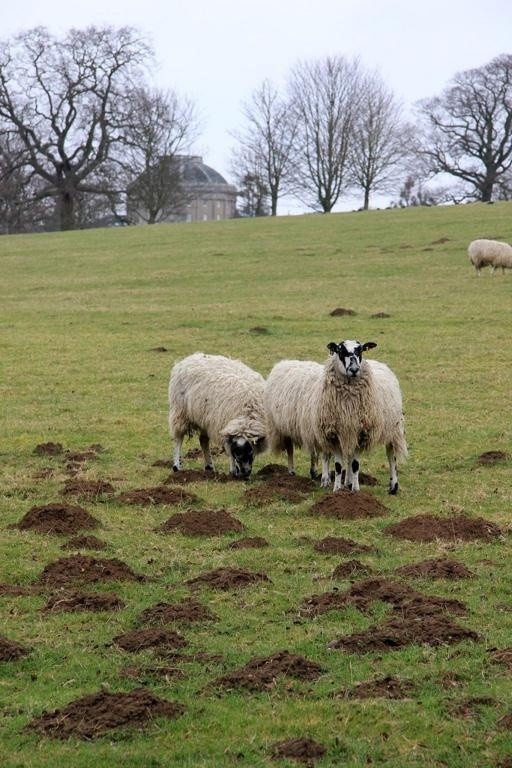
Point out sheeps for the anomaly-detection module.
[167,350,269,482]
[262,358,352,490]
[309,339,411,496]
[467,239,512,276]
